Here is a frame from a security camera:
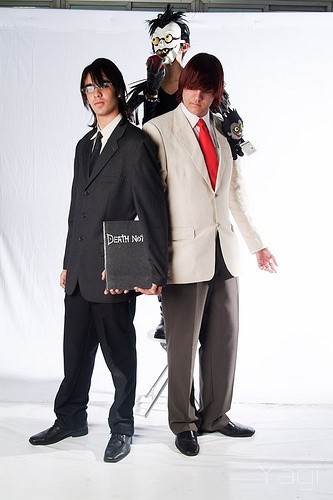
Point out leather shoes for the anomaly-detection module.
[175,430,200,456]
[29,420,89,445]
[104,433,133,463]
[198,420,255,437]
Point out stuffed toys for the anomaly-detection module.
[221,107,245,160]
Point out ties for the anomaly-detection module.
[197,119,219,192]
[89,132,103,178]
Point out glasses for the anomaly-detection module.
[80,82,114,94]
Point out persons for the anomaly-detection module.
[123,5,233,129]
[143,53,279,456]
[29,57,169,462]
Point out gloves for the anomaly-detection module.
[145,58,166,99]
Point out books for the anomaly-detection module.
[103,221,152,289]
